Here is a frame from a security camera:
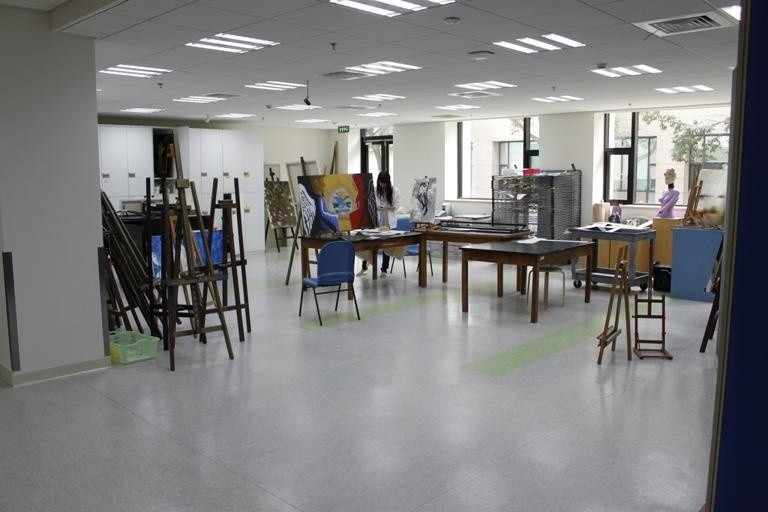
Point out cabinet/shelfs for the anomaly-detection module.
[98,123,266,258]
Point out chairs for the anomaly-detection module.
[299,240,361,326]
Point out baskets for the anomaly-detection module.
[109,330,160,364]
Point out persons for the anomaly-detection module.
[654,168,680,218]
[354,171,401,278]
